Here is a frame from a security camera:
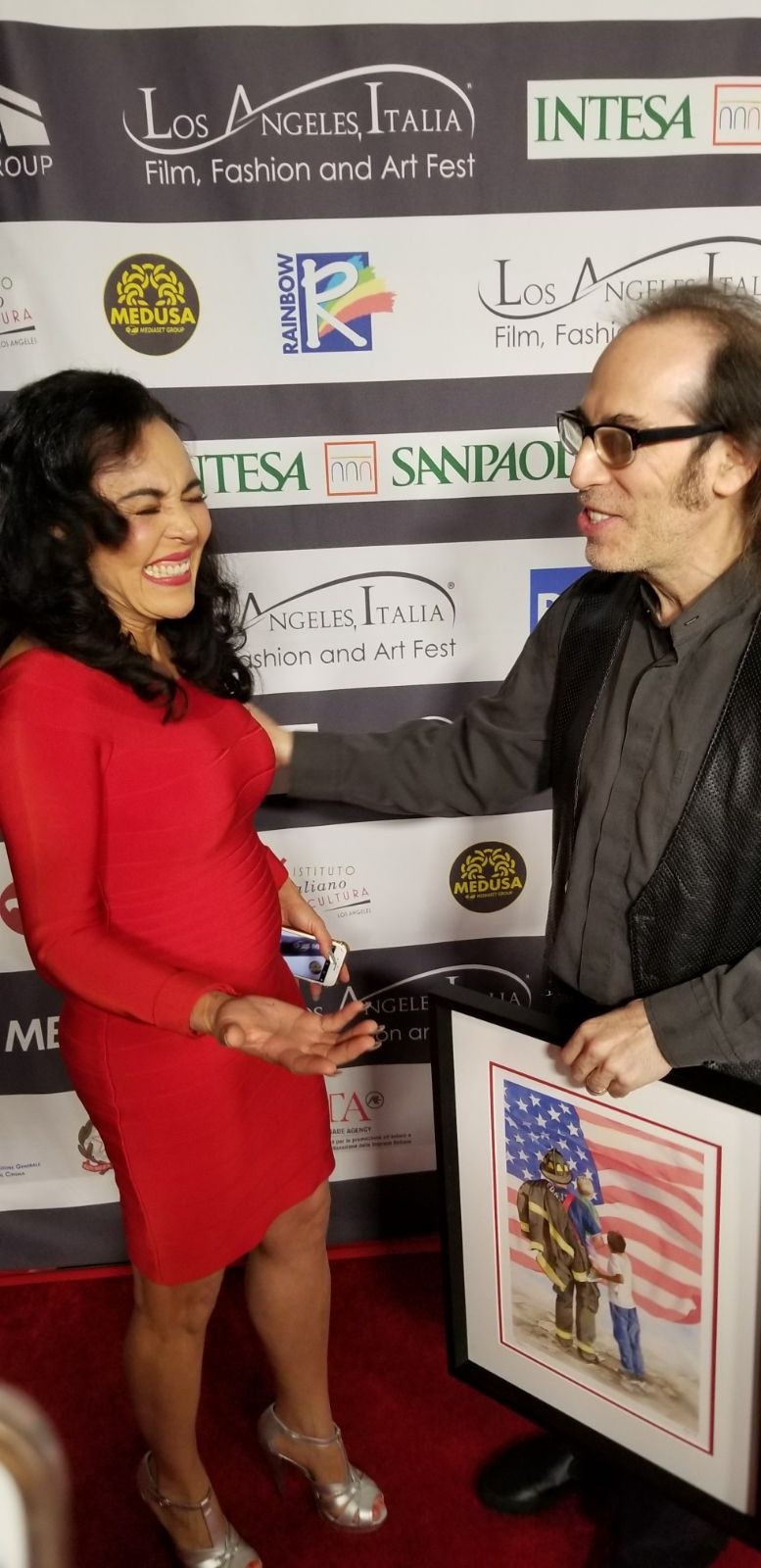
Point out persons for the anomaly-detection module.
[238,278,761,1568]
[0,368,389,1568]
[515,1149,646,1381]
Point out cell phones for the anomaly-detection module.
[278,927,349,988]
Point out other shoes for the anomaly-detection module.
[588,1488,730,1567]
[476,1431,605,1514]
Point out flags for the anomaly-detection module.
[503,1080,703,1326]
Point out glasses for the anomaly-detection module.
[556,407,728,469]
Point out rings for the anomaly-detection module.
[585,1075,608,1095]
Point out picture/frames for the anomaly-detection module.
[424,979,761,1557]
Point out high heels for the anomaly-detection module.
[136,1449,262,1568]
[256,1403,387,1533]
